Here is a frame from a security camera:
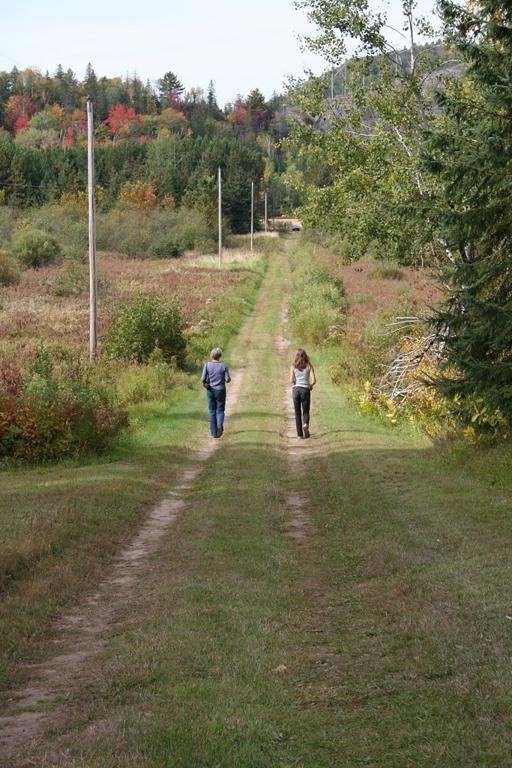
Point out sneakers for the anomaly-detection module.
[302,423,310,438]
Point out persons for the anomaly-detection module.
[289,348,316,440]
[200,348,231,439]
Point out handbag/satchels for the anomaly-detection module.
[203,381,210,388]
